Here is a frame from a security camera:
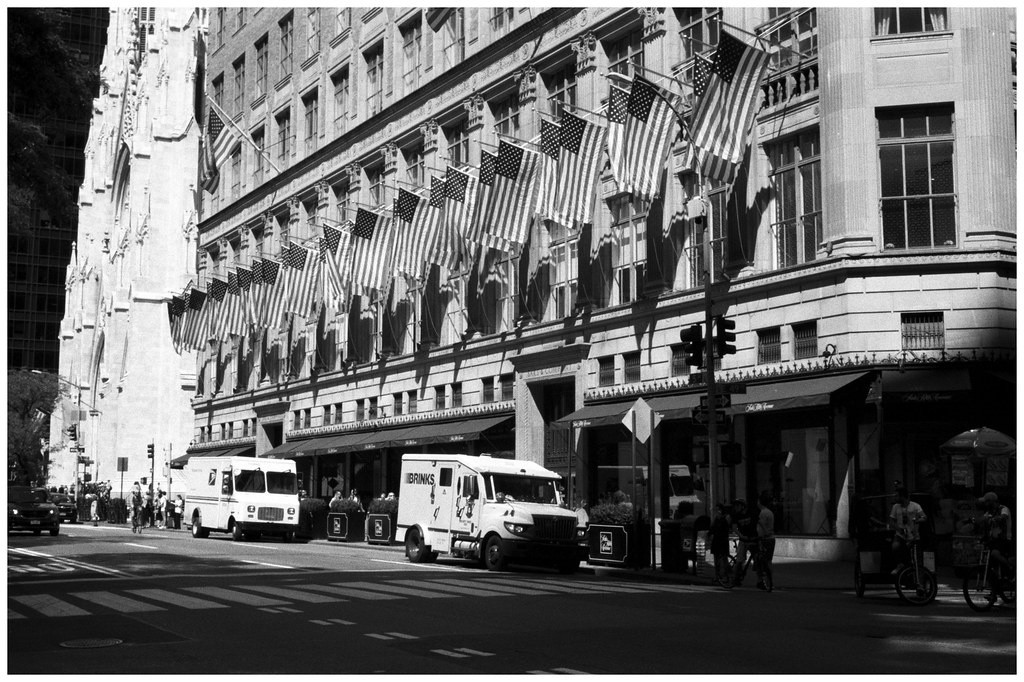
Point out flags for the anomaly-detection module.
[166,295,182,357]
[347,207,393,292]
[533,113,606,230]
[224,266,254,338]
[281,240,321,320]
[687,30,777,184]
[182,288,206,354]
[317,223,355,315]
[389,186,428,282]
[203,278,230,344]
[465,142,542,257]
[200,97,244,196]
[607,74,686,200]
[420,167,476,272]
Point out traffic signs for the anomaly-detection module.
[700,393,731,410]
[691,409,726,426]
[692,429,736,446]
[708,382,747,394]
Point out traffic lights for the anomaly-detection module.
[680,326,704,367]
[69,425,77,441]
[148,443,154,458]
[717,317,736,356]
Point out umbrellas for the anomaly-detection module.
[937,426,1016,479]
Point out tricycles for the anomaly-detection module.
[854,514,938,607]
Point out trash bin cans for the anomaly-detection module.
[167,509,175,528]
[657,518,697,572]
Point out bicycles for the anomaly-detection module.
[715,532,775,593]
[963,515,1016,613]
[131,503,144,534]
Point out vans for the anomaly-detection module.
[394,453,590,574]
[182,455,303,543]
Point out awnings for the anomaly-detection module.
[169,441,256,470]
[556,365,878,517]
[262,409,514,458]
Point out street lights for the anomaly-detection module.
[31,369,81,508]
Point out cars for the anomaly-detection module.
[7,486,61,538]
[47,492,78,524]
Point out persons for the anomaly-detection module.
[46,478,185,534]
[326,488,365,511]
[297,488,307,500]
[707,492,776,586]
[890,487,1012,601]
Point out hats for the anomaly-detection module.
[978,492,998,501]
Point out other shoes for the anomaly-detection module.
[891,568,899,575]
